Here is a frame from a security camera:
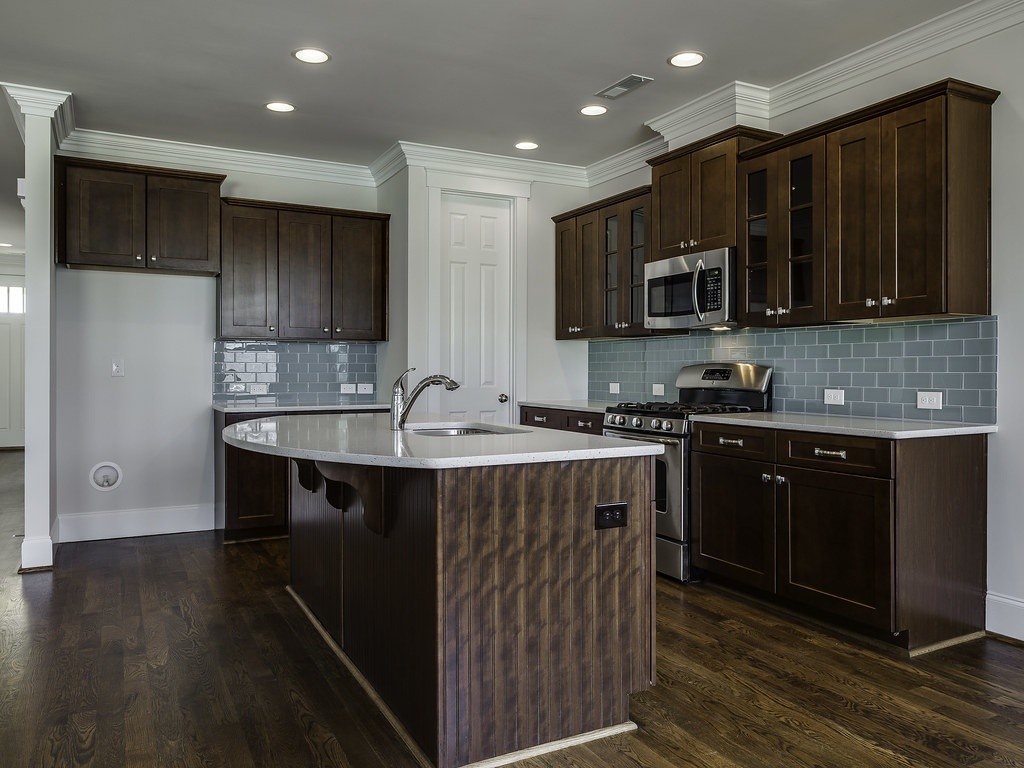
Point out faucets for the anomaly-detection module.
[387,366,461,431]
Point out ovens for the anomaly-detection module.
[603,430,688,582]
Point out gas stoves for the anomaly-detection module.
[603,363,773,433]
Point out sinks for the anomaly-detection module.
[399,421,535,437]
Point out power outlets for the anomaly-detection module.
[824,389,844,405]
[916,392,943,410]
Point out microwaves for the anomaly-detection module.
[644,249,736,332]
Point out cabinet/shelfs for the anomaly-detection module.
[54,154,227,277]
[520,406,605,435]
[551,76,1001,340]
[216,196,391,343]
[213,410,393,543]
[689,421,988,659]
[283,454,658,768]
